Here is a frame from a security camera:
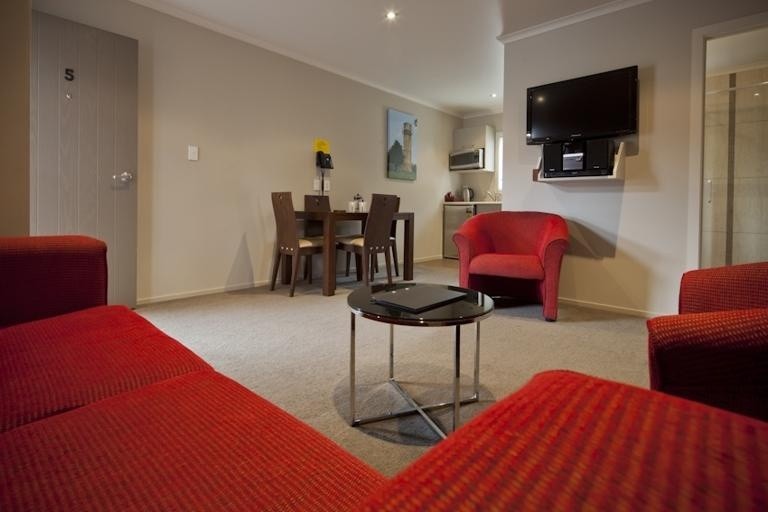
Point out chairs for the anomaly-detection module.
[269,189,418,298]
[648,260,767,419]
[453,208,570,326]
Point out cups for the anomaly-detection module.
[358,199,368,213]
[348,200,356,213]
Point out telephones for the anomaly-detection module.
[317,151,334,169]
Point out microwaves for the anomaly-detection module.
[449,147,484,170]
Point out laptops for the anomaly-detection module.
[369,285,469,314]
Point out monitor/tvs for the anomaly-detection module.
[526,65,639,145]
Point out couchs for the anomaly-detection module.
[0,231,768,512]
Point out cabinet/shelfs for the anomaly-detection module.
[443,202,502,261]
[453,124,496,174]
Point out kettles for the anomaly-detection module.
[461,185,474,202]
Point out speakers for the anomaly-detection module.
[543,143,562,173]
[586,137,614,169]
